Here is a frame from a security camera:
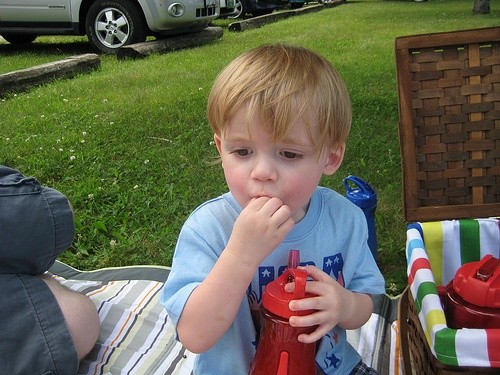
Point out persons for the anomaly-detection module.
[0,164,101,375]
[158,42,385,375]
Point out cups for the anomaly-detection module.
[444,255,500,329]
[249,250,318,374]
[343,176,377,260]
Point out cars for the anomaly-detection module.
[227,0,290,19]
[220,0,236,18]
[0,0,220,55]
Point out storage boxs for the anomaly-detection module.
[395,26,500,375]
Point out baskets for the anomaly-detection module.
[394,24,500,374]
